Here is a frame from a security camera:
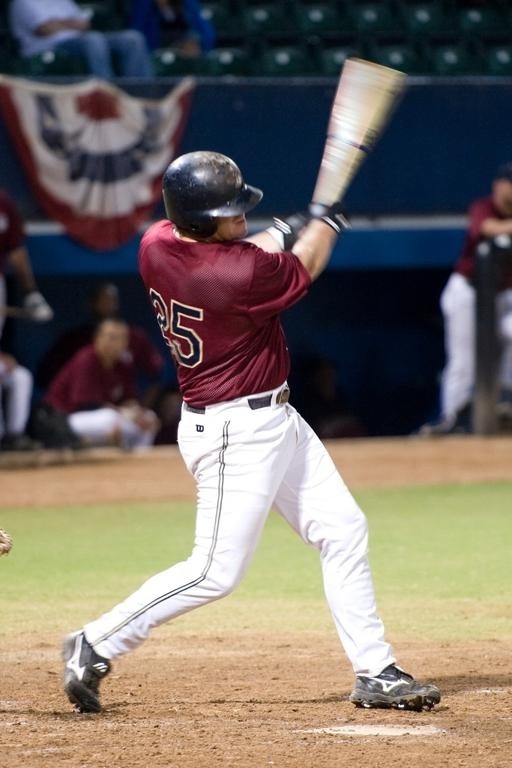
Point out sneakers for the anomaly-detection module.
[350,664,442,710]
[61,630,111,712]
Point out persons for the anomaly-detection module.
[132,0,216,76]
[1,189,53,332]
[422,169,511,433]
[63,148,441,712]
[11,0,153,77]
[2,353,48,451]
[82,282,179,445]
[44,317,161,447]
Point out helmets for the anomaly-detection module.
[163,152,265,239]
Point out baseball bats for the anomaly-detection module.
[308,58,406,216]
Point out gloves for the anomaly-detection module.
[279,202,351,249]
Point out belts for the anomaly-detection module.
[183,385,290,414]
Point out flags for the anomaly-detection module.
[2,75,197,249]
